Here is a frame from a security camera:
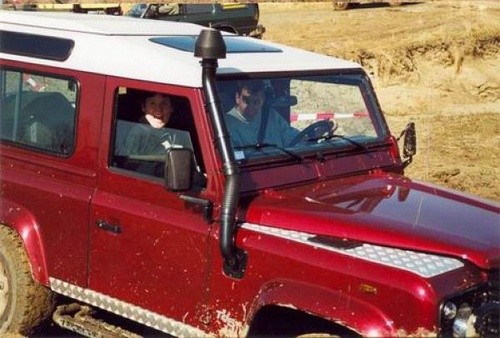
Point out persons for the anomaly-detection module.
[224,79,337,149]
[125,90,187,162]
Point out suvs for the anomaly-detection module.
[0,9,500,338]
[128,2,265,40]
